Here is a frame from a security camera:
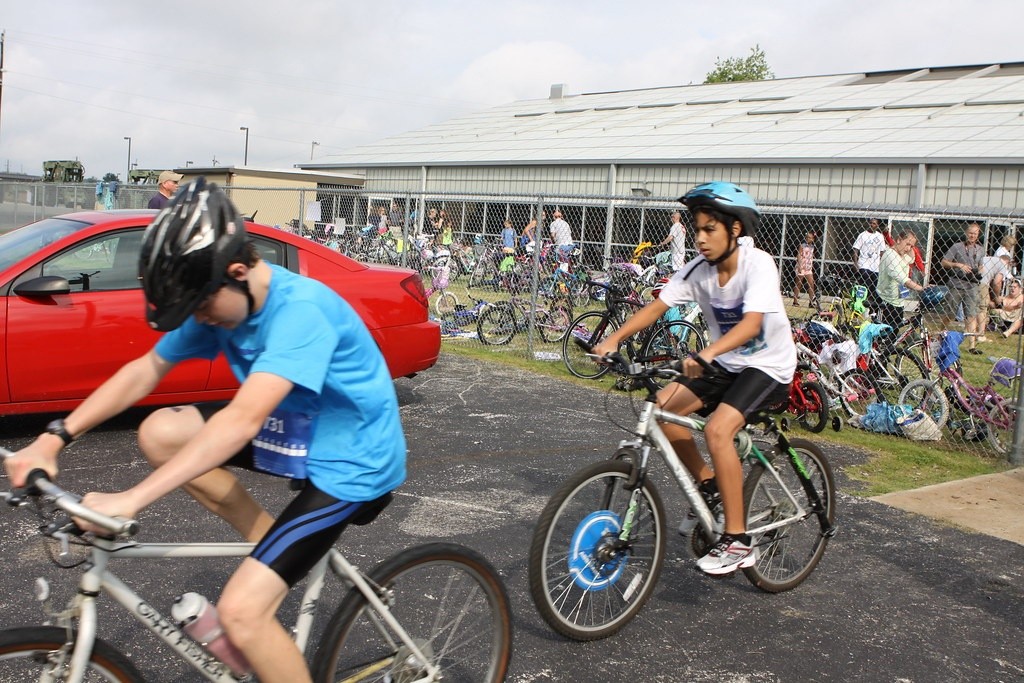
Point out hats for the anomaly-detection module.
[158,171,184,184]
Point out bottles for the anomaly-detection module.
[170,593,251,677]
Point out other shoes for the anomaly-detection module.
[970,348,983,355]
[792,302,800,307]
[809,304,818,308]
[1003,332,1008,339]
[880,383,894,390]
[976,336,992,343]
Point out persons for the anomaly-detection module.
[853,217,1024,377]
[661,212,687,272]
[591,181,797,573]
[148,169,184,208]
[793,230,816,307]
[0,177,407,683]
[367,200,574,263]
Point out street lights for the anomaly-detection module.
[310,141,320,159]
[240,126,249,166]
[124,136,131,170]
[186,161,193,167]
[131,163,138,169]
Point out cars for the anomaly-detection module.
[0,207,441,416]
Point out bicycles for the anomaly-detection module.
[528,340,835,642]
[0,445,514,683]
[288,218,1022,457]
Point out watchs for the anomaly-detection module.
[47,418,73,446]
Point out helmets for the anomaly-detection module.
[139,176,246,331]
[676,181,762,236]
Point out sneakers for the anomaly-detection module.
[696,533,756,575]
[678,477,723,537]
